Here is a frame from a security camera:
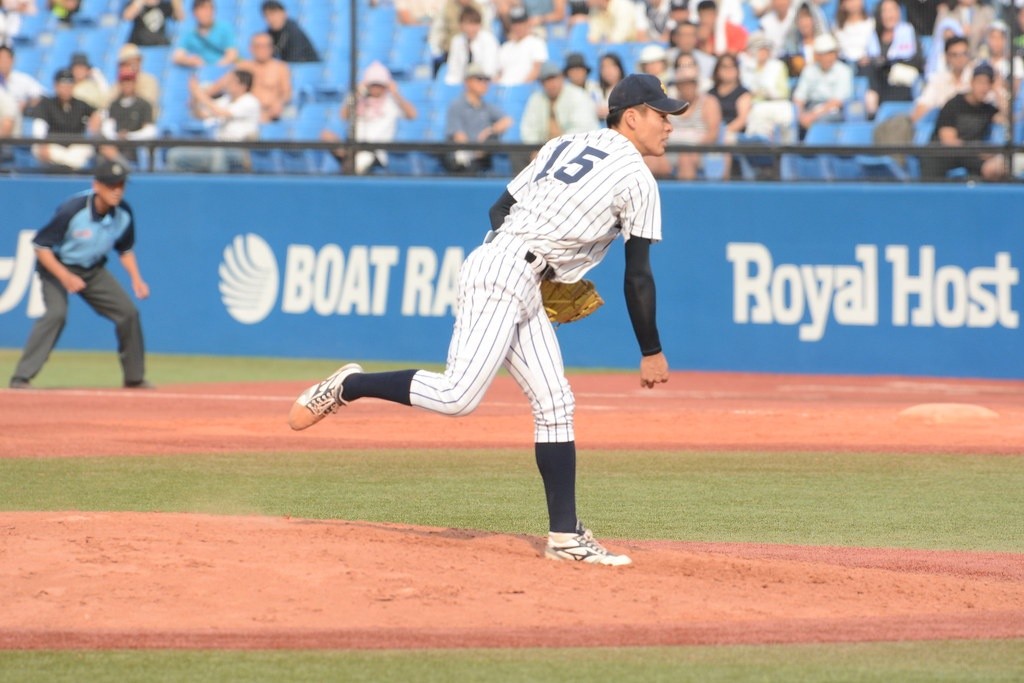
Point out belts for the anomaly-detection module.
[485,231,557,281]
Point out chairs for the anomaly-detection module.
[0,0,1024,180]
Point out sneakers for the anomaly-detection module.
[544,519,632,567]
[289,363,365,431]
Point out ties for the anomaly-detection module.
[467,39,472,64]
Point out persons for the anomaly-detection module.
[288,73,690,567]
[9,160,156,388]
[0,0,1024,181]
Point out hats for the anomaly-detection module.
[668,1,1023,85]
[640,45,667,64]
[72,54,91,68]
[537,61,560,81]
[117,64,136,81]
[55,70,75,84]
[508,6,530,23]
[119,43,142,63]
[608,74,690,116]
[464,61,491,82]
[95,161,128,185]
[563,54,591,74]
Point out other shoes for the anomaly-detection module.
[10,376,34,390]
[124,381,155,389]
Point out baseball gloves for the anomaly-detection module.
[539,277,605,324]
